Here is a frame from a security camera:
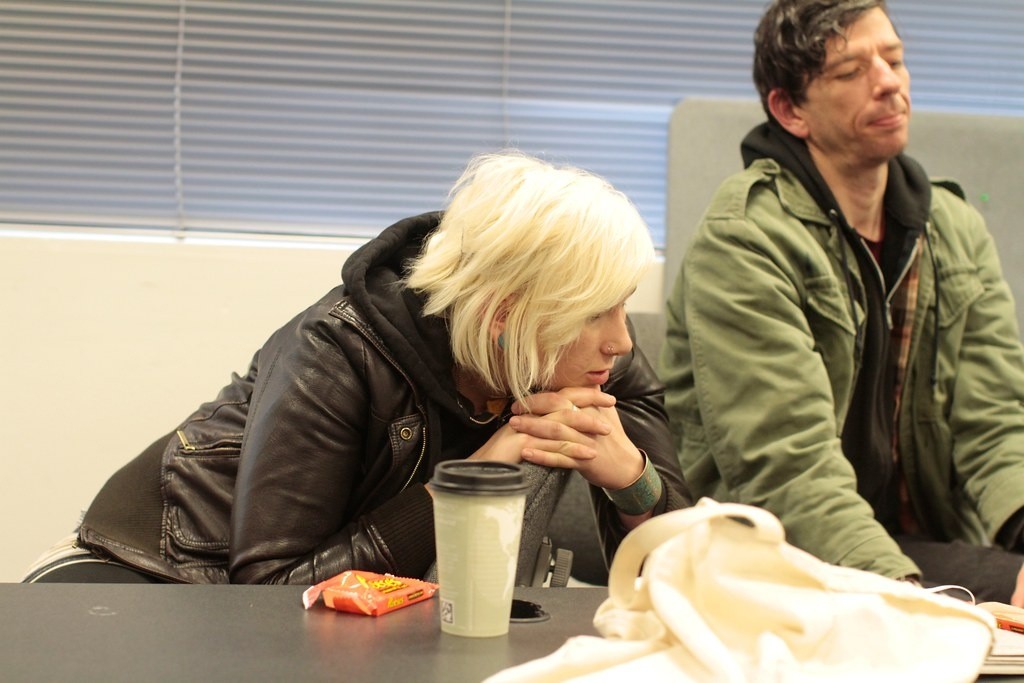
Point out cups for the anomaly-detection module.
[425,458,532,639]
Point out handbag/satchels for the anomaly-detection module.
[486,496,996,683]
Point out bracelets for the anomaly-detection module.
[602,446,663,516]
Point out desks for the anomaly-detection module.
[0,583,609,683]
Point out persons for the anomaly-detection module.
[16,151,693,587]
[658,0,1024,609]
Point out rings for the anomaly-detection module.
[572,405,577,411]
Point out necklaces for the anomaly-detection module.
[470,414,500,424]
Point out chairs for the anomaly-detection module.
[663,96,1024,345]
